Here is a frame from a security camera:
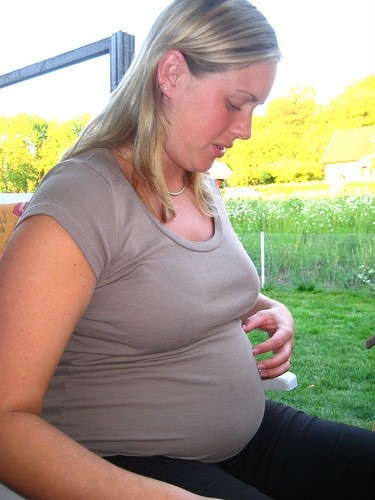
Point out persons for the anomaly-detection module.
[0,1,374,500]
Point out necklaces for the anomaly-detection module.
[113,143,187,197]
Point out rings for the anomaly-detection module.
[287,357,292,364]
